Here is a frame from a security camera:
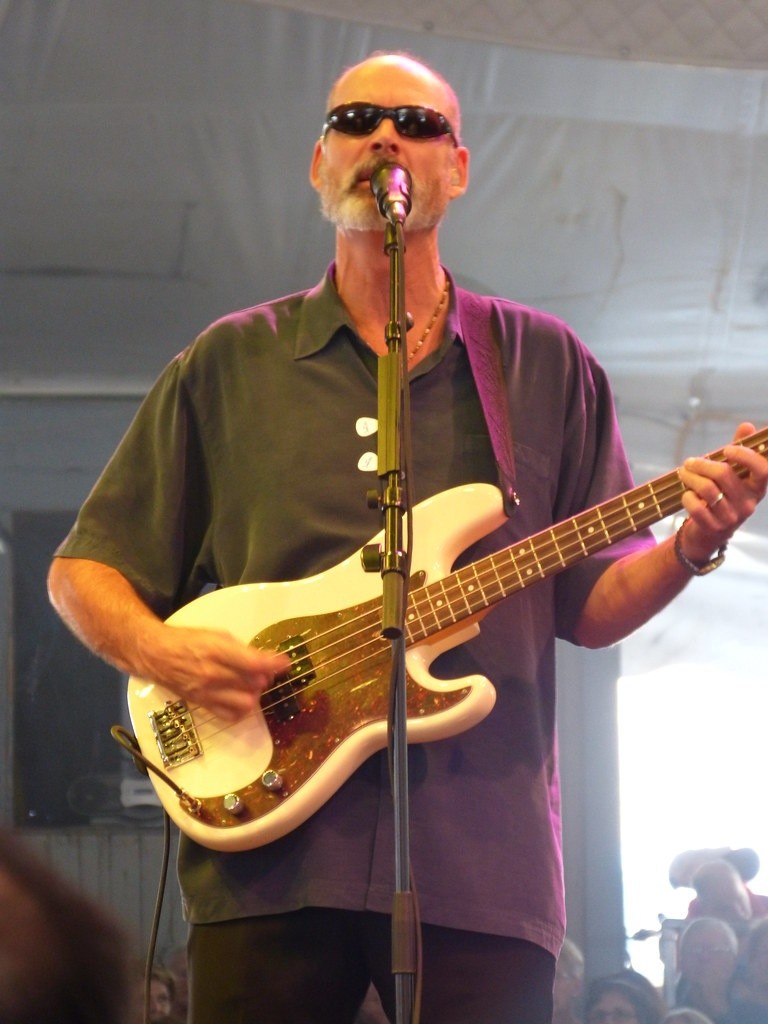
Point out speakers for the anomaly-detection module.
[12,508,175,832]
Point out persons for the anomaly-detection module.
[45,51,768,1024]
[553,847,768,1024]
[0,832,196,1024]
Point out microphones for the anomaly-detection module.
[369,162,412,227]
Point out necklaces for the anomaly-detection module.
[399,276,451,370]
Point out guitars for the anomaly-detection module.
[127,422,767,856]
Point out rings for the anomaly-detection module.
[710,492,725,507]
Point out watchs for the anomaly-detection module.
[666,521,728,577]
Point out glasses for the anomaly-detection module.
[321,100,463,149]
[681,943,736,958]
